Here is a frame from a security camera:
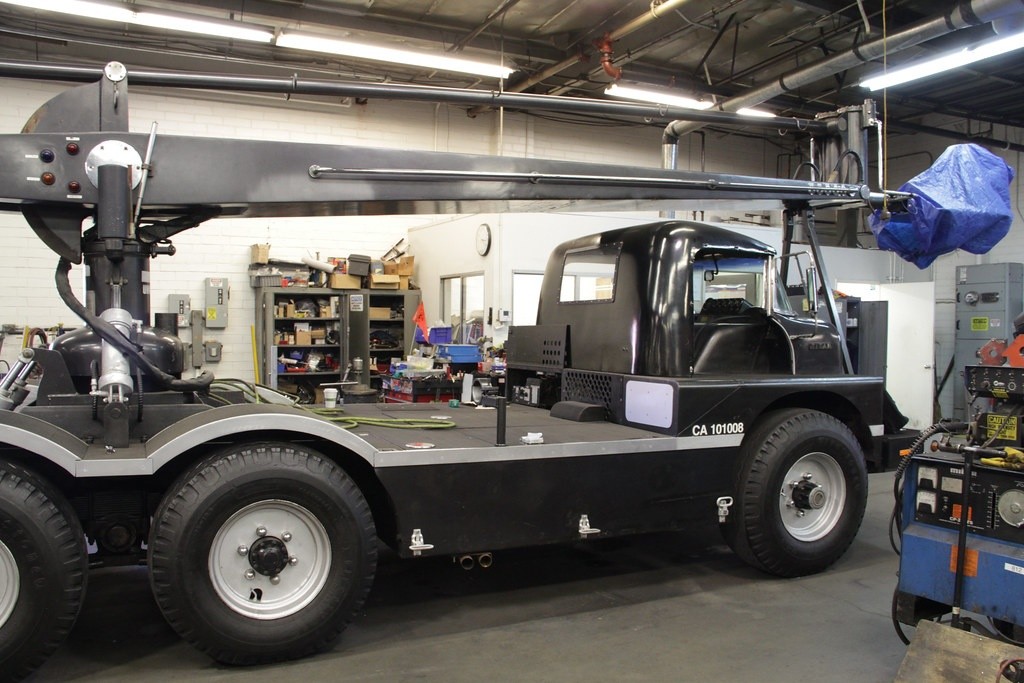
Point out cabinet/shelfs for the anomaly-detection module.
[790,262,1023,471]
[255,290,423,406]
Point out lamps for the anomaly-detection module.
[0,1,1024,116]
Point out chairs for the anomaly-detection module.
[700,297,754,315]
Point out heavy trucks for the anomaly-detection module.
[1,58,1016,680]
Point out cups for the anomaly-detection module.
[353,357,363,371]
[449,400,459,407]
[323,388,338,409]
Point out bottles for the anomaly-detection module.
[477,338,508,375]
[324,353,334,369]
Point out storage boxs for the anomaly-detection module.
[315,339,325,344]
[324,388,338,399]
[436,345,483,363]
[290,350,303,359]
[277,384,297,393]
[314,388,325,403]
[279,308,284,317]
[415,326,452,344]
[295,331,312,345]
[370,307,391,320]
[327,255,414,290]
[286,304,295,317]
[250,275,285,287]
[250,242,271,265]
[311,330,326,338]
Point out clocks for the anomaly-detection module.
[476,223,491,256]
